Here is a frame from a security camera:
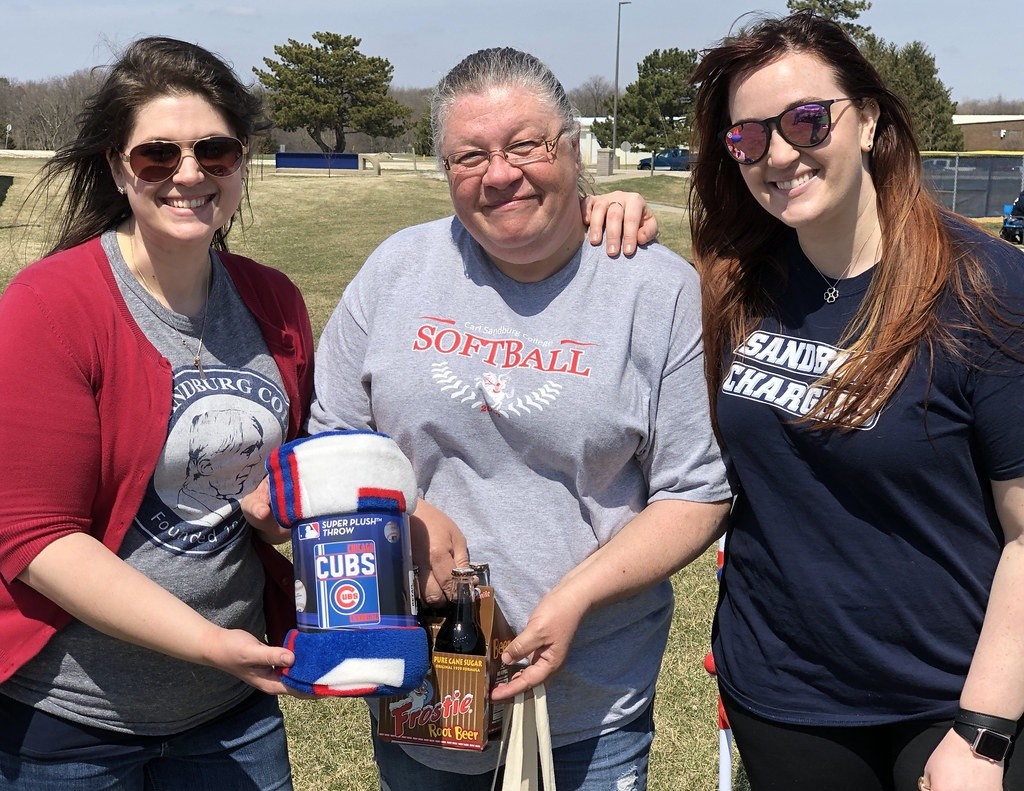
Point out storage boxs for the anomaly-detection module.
[376,584,517,756]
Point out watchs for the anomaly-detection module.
[952,721,1012,762]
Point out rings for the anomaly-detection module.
[607,202,625,212]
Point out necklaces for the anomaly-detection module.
[796,220,878,303]
[128,217,209,380]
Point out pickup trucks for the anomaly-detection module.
[637,149,698,171]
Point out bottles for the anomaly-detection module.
[413,565,433,666]
[433,567,486,656]
[469,563,488,586]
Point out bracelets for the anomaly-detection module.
[955,707,1017,737]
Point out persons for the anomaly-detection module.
[307,48,732,791]
[581,8,1024,791]
[0,37,328,791]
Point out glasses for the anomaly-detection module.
[113,133,248,184]
[440,126,567,173]
[717,96,861,166]
